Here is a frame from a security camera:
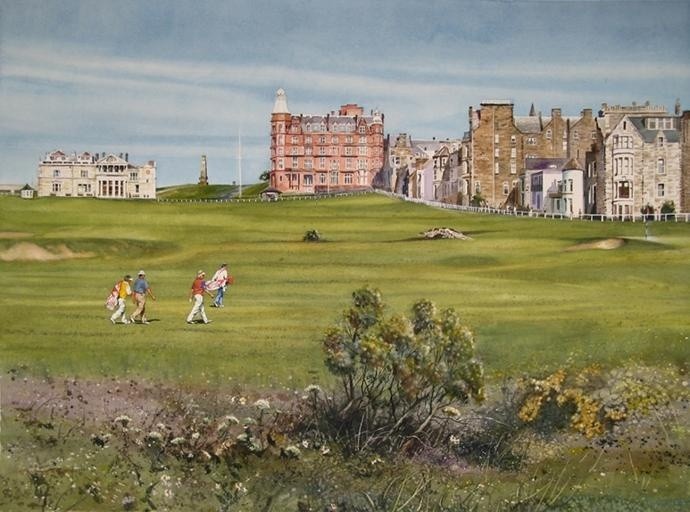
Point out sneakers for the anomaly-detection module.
[109,317,116,324]
[121,317,136,324]
[205,320,213,325]
[212,300,226,308]
[141,320,150,326]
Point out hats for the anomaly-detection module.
[138,270,147,275]
[123,278,134,281]
[196,269,206,278]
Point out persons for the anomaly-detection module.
[209,261,229,308]
[109,272,135,325]
[129,269,157,326]
[185,269,214,324]
